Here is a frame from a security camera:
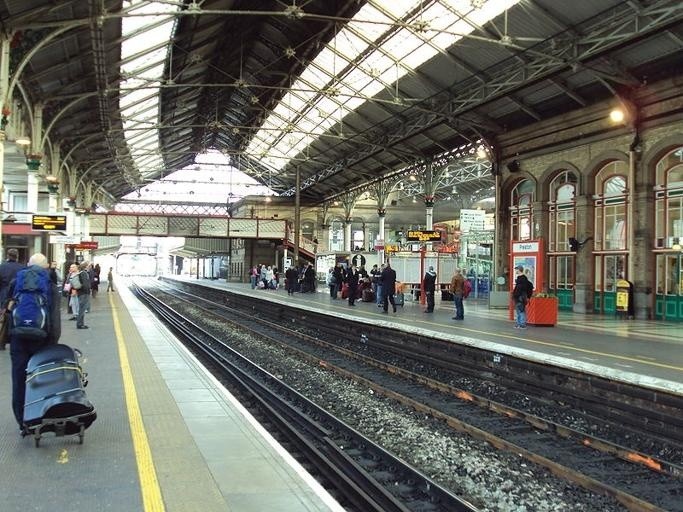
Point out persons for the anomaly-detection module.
[449,267,465,321]
[354,244,378,252]
[1,248,27,351]
[423,265,438,313]
[499,267,511,291]
[249,262,385,308]
[313,235,318,253]
[512,265,529,330]
[380,262,397,314]
[49,259,116,330]
[1,252,63,437]
[525,269,531,281]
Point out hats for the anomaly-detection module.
[513,264,523,271]
[26,253,49,269]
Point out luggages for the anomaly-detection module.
[391,281,405,307]
[22,342,96,448]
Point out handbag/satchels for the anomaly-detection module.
[62,283,72,292]
[462,278,472,299]
[361,286,375,302]
[0,302,12,349]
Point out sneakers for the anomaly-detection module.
[18,426,28,436]
[69,315,89,329]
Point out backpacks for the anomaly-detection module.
[12,265,51,343]
[525,278,533,298]
[70,270,86,290]
